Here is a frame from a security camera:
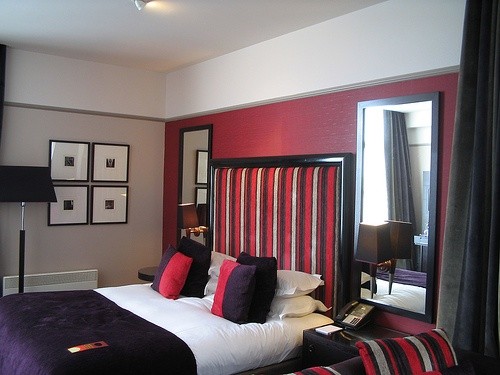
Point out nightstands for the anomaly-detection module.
[302,322,412,367]
[137,266,160,283]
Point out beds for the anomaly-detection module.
[0,152,354,375]
[360,267,427,313]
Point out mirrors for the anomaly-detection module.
[177,123,212,249]
[350,92,441,324]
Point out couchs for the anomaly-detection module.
[283,351,475,375]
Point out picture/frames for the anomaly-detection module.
[47,183,89,226]
[91,143,129,183]
[48,139,91,182]
[90,184,130,225]
[195,150,208,204]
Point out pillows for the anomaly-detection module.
[283,329,459,375]
[149,237,333,319]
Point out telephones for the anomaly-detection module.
[334,300,375,329]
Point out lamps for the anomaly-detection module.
[197,204,206,239]
[0,165,57,292]
[178,202,199,239]
[386,219,413,293]
[134,0,150,10]
[354,221,390,299]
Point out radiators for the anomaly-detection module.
[3,269,98,296]
